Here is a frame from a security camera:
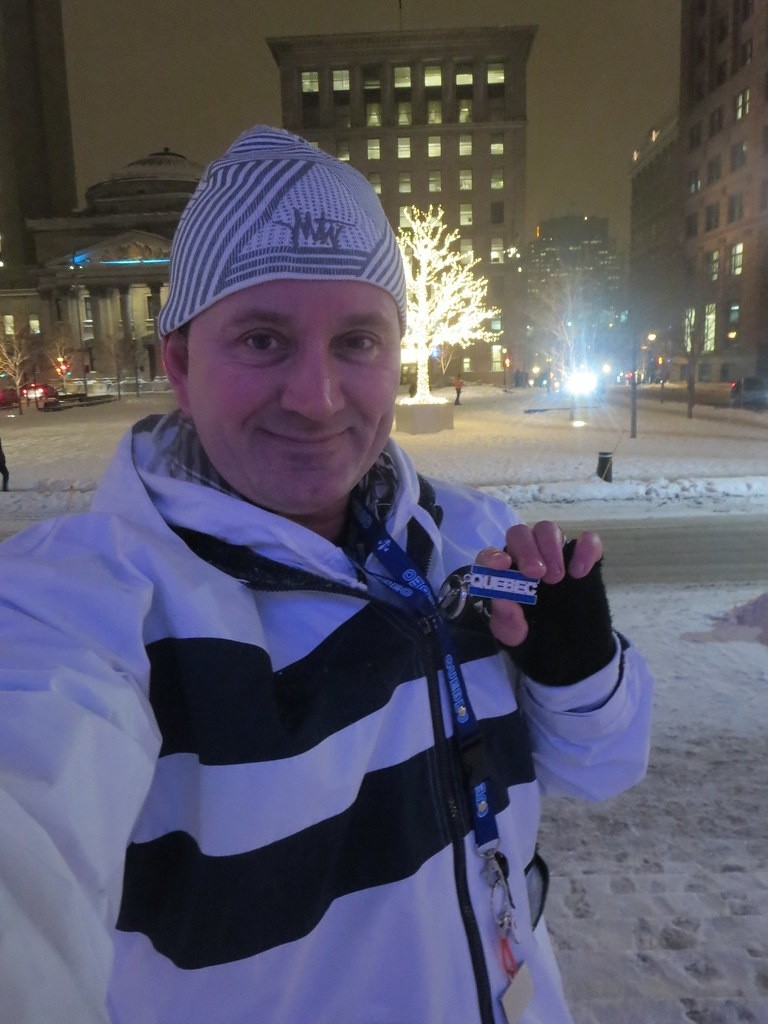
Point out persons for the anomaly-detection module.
[0,125,654,1023]
[409,365,644,406]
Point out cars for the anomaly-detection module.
[0,388,21,409]
[729,376,768,412]
[23,384,59,400]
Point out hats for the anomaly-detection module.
[158,124,408,341]
